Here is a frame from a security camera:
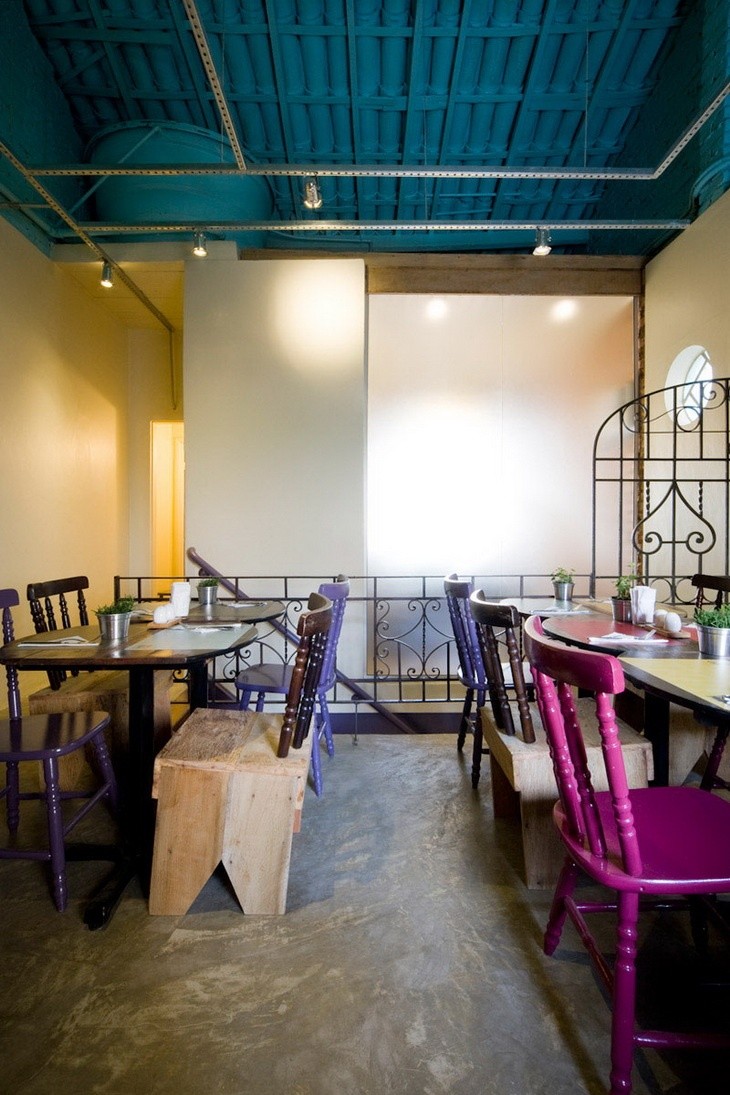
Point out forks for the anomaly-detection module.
[176,619,234,630]
[590,630,656,641]
[528,604,582,613]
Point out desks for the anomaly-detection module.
[541,615,700,787]
[128,599,283,713]
[616,647,730,719]
[499,594,688,620]
[0,619,259,931]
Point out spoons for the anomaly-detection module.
[20,639,84,646]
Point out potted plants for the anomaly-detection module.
[612,562,644,622]
[91,599,132,640]
[693,603,730,656]
[196,579,218,604]
[549,566,576,602]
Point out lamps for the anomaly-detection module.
[533,226,552,257]
[100,256,115,289]
[302,174,323,209]
[192,231,208,257]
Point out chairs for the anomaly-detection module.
[690,573,730,611]
[0,587,126,914]
[442,573,537,791]
[232,573,350,799]
[523,614,729,1095]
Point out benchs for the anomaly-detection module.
[466,589,651,892]
[147,590,334,918]
[24,574,177,800]
